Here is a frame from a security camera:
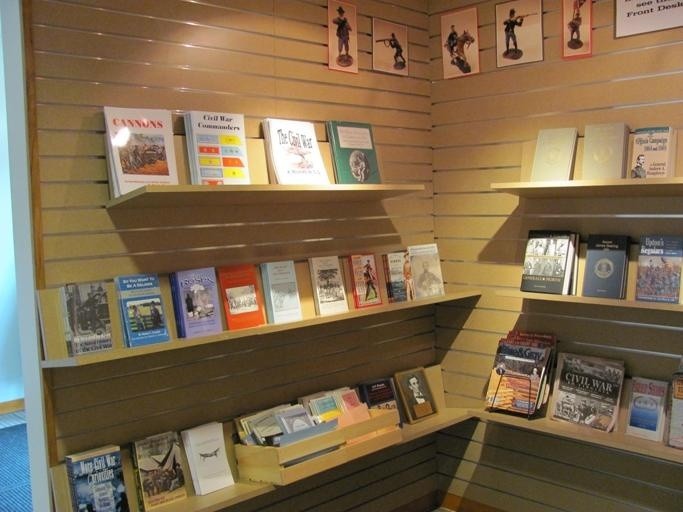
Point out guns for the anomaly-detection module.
[375,37,395,47]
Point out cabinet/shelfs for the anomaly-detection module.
[468,178,683,464]
[41,183,483,512]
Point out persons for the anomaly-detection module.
[390,33,406,64]
[357,156,366,182]
[570,13,581,40]
[503,9,523,53]
[448,25,458,55]
[144,463,184,497]
[334,7,351,61]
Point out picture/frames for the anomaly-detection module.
[562,1,592,60]
[372,16,409,77]
[495,0,544,68]
[327,1,359,74]
[439,7,480,80]
[613,1,683,39]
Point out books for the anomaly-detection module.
[103,106,382,199]
[486,331,682,449]
[61,244,444,357]
[66,365,437,511]
[531,123,676,182]
[521,230,681,303]
